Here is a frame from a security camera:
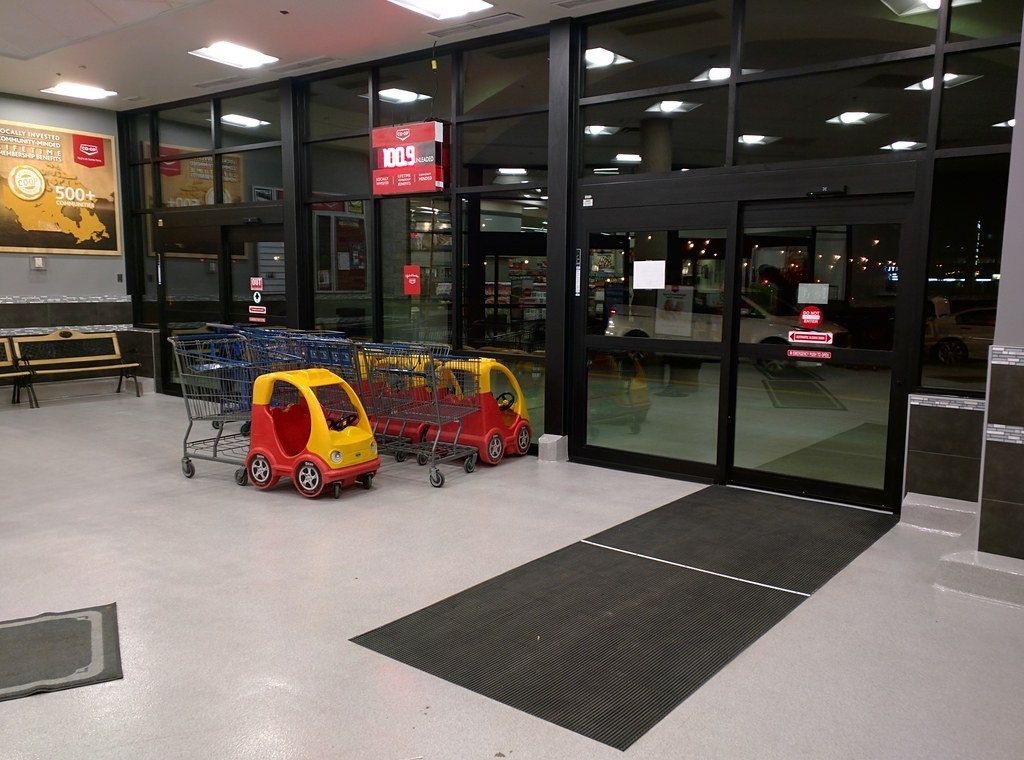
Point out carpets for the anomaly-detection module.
[347,480,900,753]
[0,602,124,701]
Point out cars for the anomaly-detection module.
[924,295,996,366]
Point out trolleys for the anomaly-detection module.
[166,330,308,488]
[203,321,484,489]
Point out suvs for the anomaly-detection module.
[604,284,852,377]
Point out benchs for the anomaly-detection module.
[0,336,35,408]
[170,325,221,375]
[10,329,143,408]
